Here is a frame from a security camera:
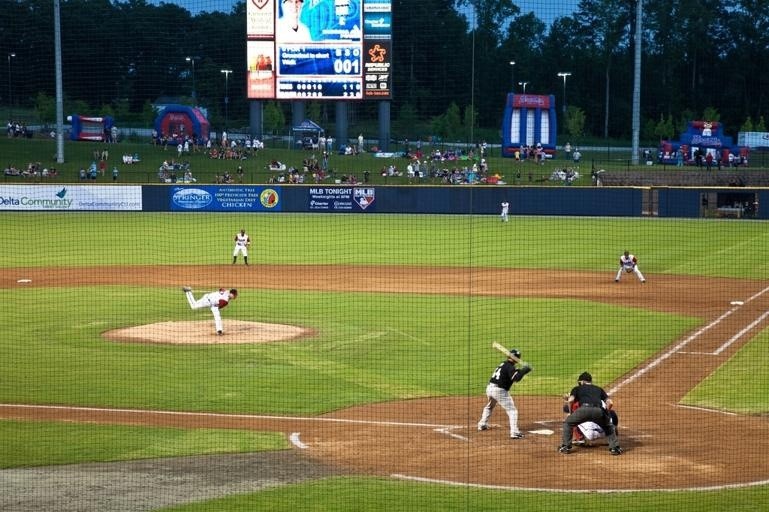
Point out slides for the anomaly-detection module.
[526,108,535,146]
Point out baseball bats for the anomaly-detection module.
[491,341,535,372]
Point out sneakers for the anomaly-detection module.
[216,331,223,335]
[478,424,490,430]
[183,286,191,291]
[572,440,586,445]
[610,446,624,455]
[558,446,568,454]
[511,433,525,440]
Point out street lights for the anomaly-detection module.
[7,52,16,120]
[185,56,195,106]
[518,81,530,94]
[557,72,572,146]
[509,61,517,92]
[220,69,232,140]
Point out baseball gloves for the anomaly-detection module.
[562,392,571,403]
[626,267,633,274]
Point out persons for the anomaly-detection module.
[1,119,769,187]
[275,0,311,43]
[232,228,251,266]
[562,391,620,446]
[557,371,624,455]
[182,285,238,336]
[501,199,511,223]
[614,252,646,283]
[474,349,533,439]
[701,194,708,218]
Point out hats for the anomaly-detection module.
[578,372,592,382]
[230,290,237,293]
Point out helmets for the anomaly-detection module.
[507,350,521,362]
